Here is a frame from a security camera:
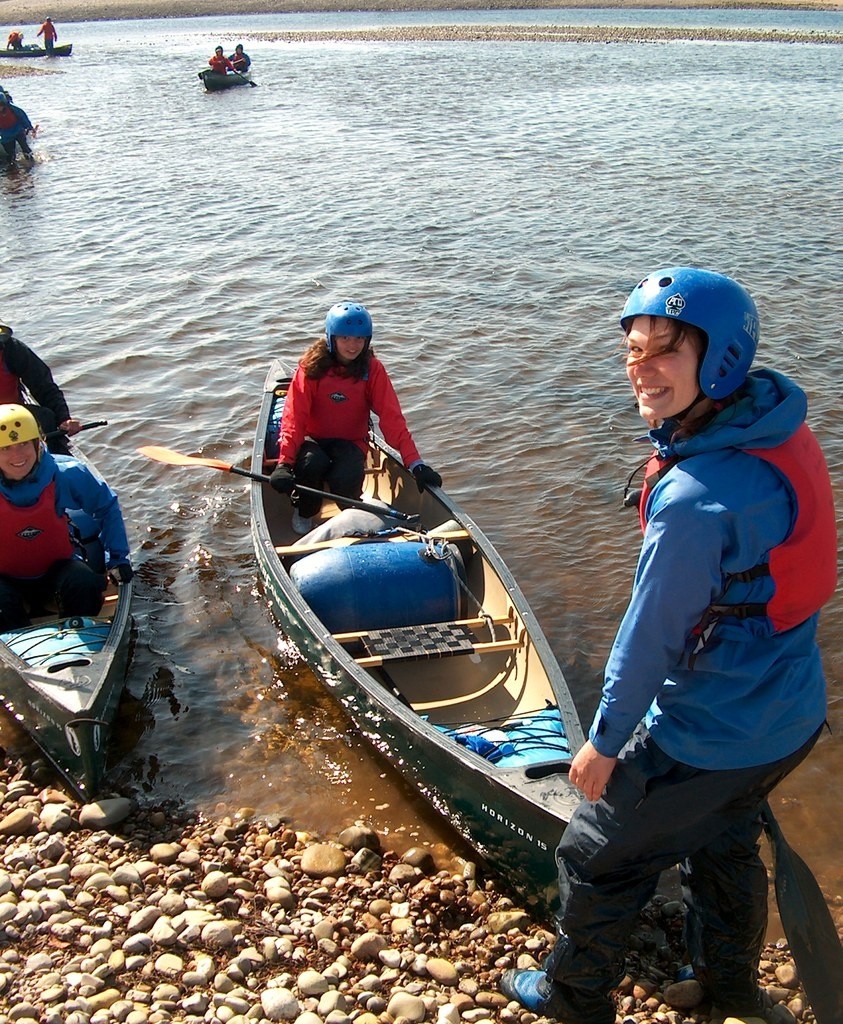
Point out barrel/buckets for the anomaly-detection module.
[290,538,468,656]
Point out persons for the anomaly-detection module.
[37,17,57,56]
[209,46,238,75]
[0,87,36,166]
[269,303,441,535]
[0,327,82,456]
[0,404,134,636]
[226,44,251,71]
[7,32,24,50]
[498,267,838,1024]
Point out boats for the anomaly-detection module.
[203,68,251,91]
[248,358,587,924]
[0,436,133,806]
[0,44,73,57]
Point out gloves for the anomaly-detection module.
[413,464,443,494]
[108,563,134,587]
[270,464,296,495]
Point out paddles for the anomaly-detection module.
[198,60,242,80]
[222,61,258,86]
[133,444,421,523]
[47,418,108,439]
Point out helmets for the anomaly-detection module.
[236,44,243,53]
[47,17,51,22]
[215,46,223,57]
[0,403,41,463]
[619,267,761,398]
[0,85,8,107]
[18,33,24,40]
[325,301,372,355]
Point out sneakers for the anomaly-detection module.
[675,965,767,1015]
[292,506,312,534]
[500,966,617,1024]
